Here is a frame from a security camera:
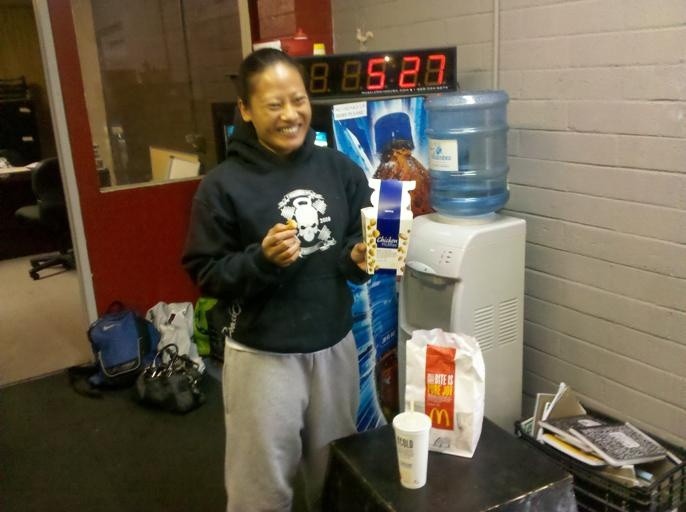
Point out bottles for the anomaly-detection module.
[105,125,134,183]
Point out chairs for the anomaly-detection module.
[14,155,76,280]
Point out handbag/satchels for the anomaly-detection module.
[146,298,218,376]
[131,343,206,415]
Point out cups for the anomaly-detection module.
[391,409,435,490]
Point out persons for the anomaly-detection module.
[176,46,377,512]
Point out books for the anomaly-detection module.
[531,379,685,512]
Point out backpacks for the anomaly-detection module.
[88,299,161,390]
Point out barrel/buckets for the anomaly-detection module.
[424,90,510,217]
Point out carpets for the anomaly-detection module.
[1,358,226,511]
